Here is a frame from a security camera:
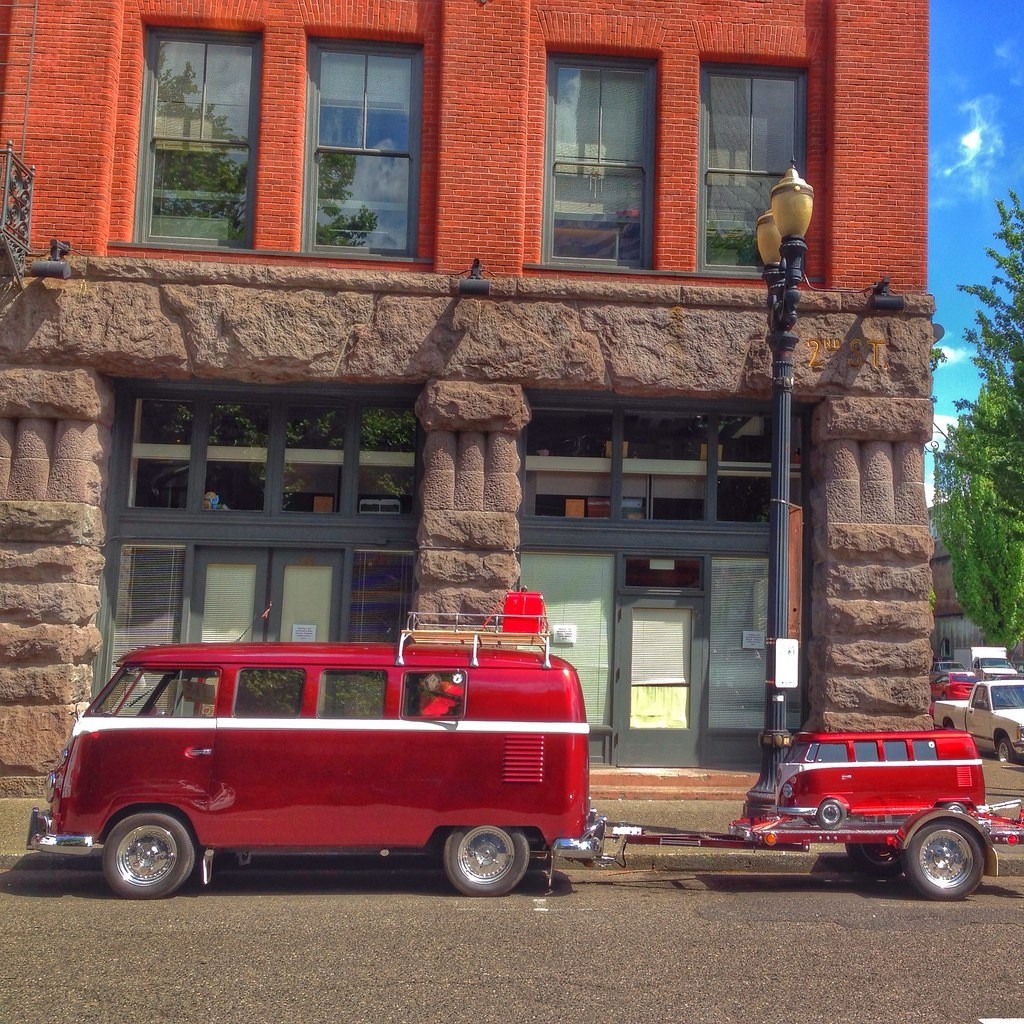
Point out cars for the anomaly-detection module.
[931,661,973,684]
[930,673,984,717]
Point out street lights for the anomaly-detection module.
[752,160,815,827]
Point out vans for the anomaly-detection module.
[31,597,594,899]
[953,646,1018,685]
[772,729,986,832]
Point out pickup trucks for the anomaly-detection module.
[933,680,1024,766]
